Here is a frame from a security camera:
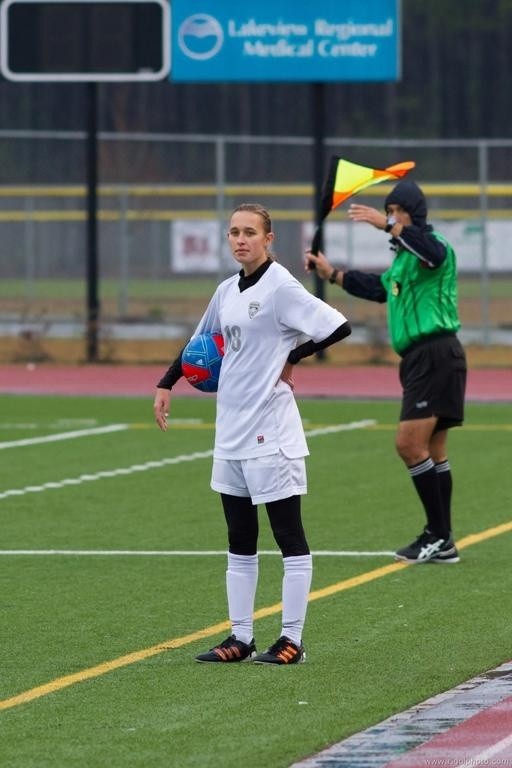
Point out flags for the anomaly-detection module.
[325,158,415,210]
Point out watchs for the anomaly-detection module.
[383,218,397,233]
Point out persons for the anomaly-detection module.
[153,202,352,667]
[303,179,468,565]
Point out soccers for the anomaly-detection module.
[182,335,223,393]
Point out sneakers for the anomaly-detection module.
[253,636,306,665]
[194,635,257,663]
[394,525,461,563]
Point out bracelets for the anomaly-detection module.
[328,268,338,284]
